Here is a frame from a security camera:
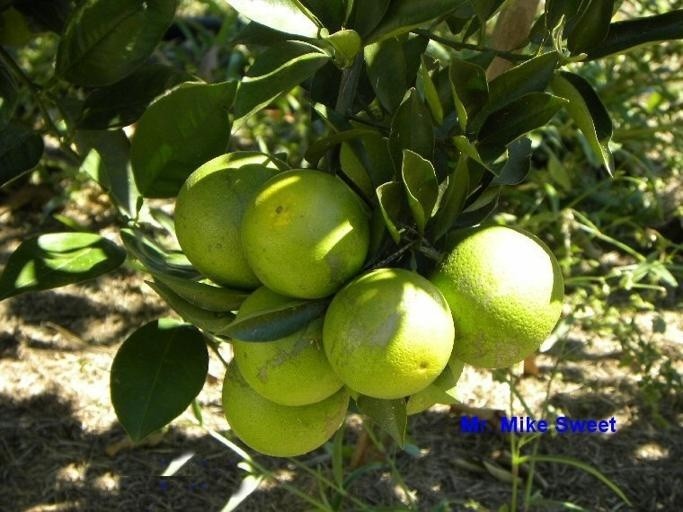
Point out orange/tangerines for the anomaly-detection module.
[344,385,433,416]
[429,222,565,369]
[222,358,350,458]
[232,285,345,407]
[240,169,372,300]
[173,151,293,291]
[322,266,456,401]
